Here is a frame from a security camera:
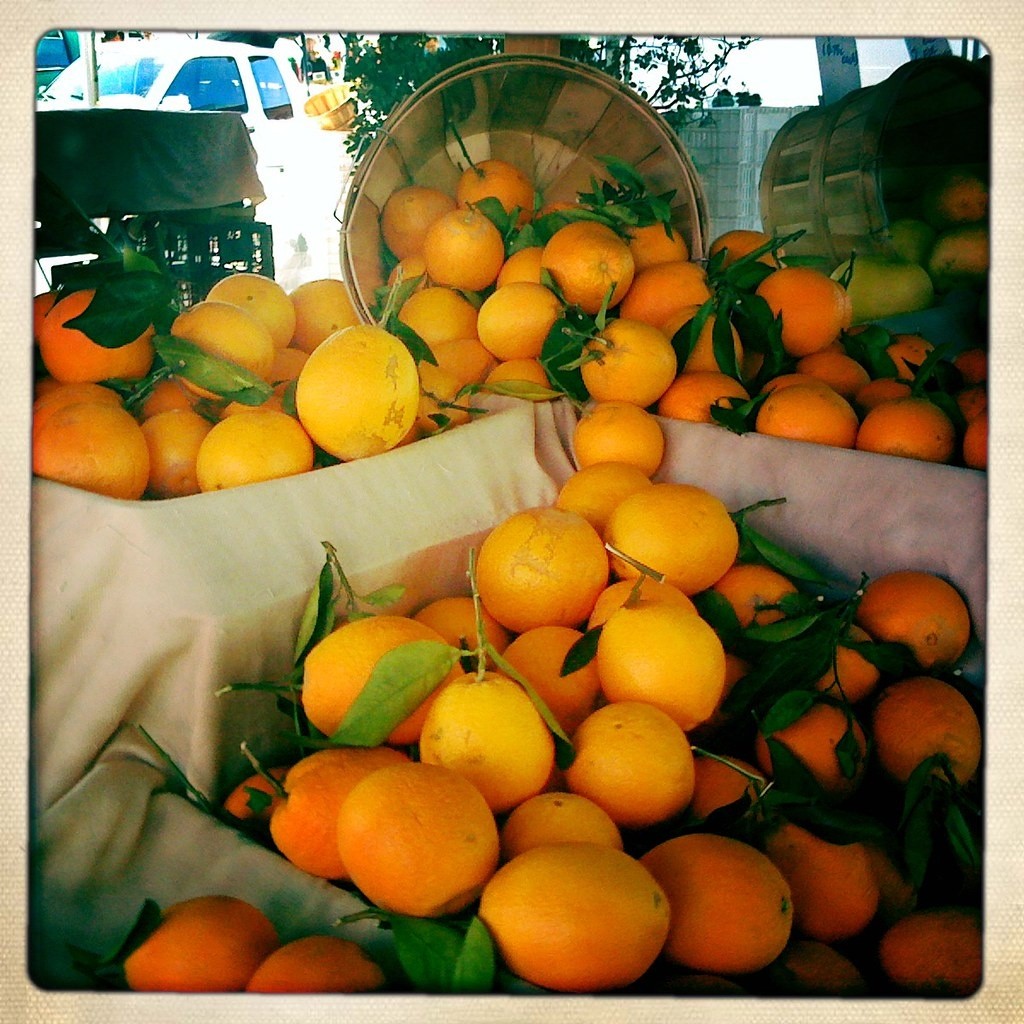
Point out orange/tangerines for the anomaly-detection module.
[125,894,387,994]
[375,160,870,454]
[220,400,985,999]
[32,272,421,501]
[830,159,989,320]
[847,331,988,474]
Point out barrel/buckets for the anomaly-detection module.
[757,54,991,281]
[332,56,711,410]
[302,83,358,132]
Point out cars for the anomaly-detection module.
[35,28,314,131]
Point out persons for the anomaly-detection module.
[424,35,449,60]
[294,34,335,86]
[101,29,124,43]
